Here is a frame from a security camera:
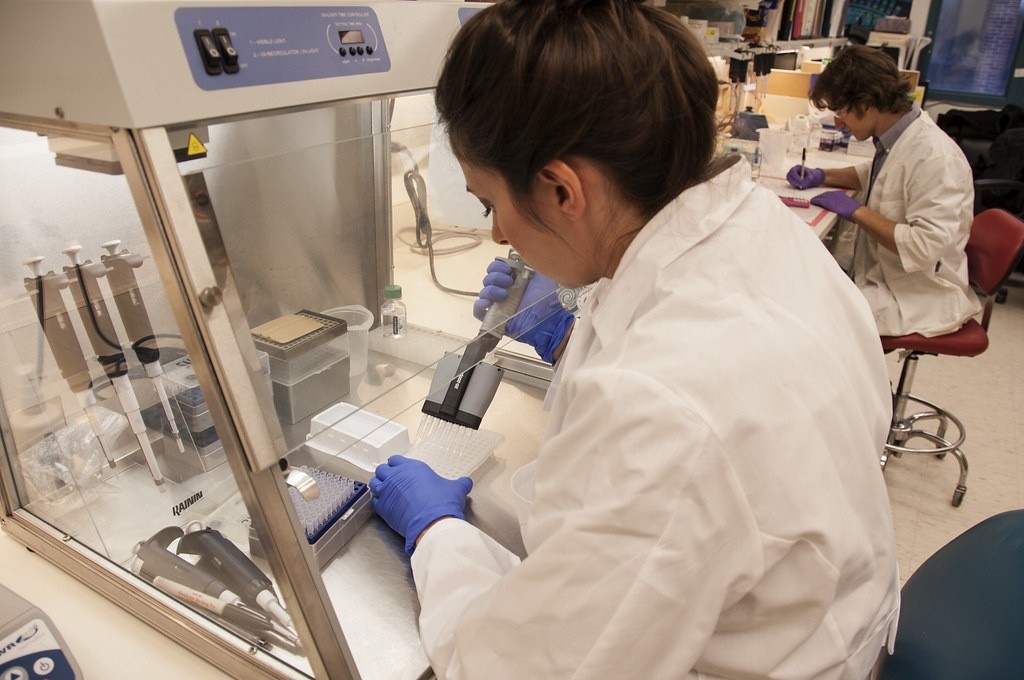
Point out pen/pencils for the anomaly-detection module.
[799,148,807,190]
[154,574,301,653]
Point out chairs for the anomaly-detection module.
[974,128,1023,305]
[873,207,1024,506]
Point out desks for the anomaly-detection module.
[0,123,872,680]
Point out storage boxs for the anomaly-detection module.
[820,130,842,153]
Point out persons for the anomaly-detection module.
[365,1,901,679]
[786,44,984,338]
